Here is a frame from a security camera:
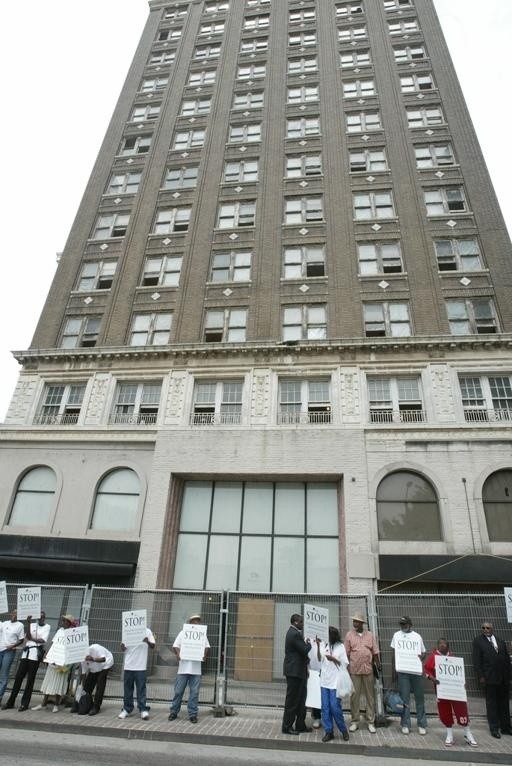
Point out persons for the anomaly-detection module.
[342,609,383,733]
[73,643,115,716]
[281,613,313,736]
[1,610,51,711]
[389,613,428,735]
[423,636,480,748]
[471,621,512,740]
[30,613,74,714]
[47,616,82,700]
[168,613,210,723]
[313,624,351,742]
[0,608,27,707]
[118,624,156,721]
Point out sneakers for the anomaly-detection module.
[2,703,77,713]
[118,708,128,718]
[446,735,454,745]
[464,735,477,745]
[282,717,427,740]
[141,711,149,718]
[191,717,197,722]
[168,713,176,720]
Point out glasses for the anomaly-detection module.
[482,626,491,630]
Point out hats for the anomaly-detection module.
[62,613,74,623]
[348,611,368,623]
[187,613,202,625]
[399,616,411,625]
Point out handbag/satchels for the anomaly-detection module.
[383,688,405,716]
[78,689,93,715]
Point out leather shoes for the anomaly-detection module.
[89,702,99,715]
[491,730,500,738]
